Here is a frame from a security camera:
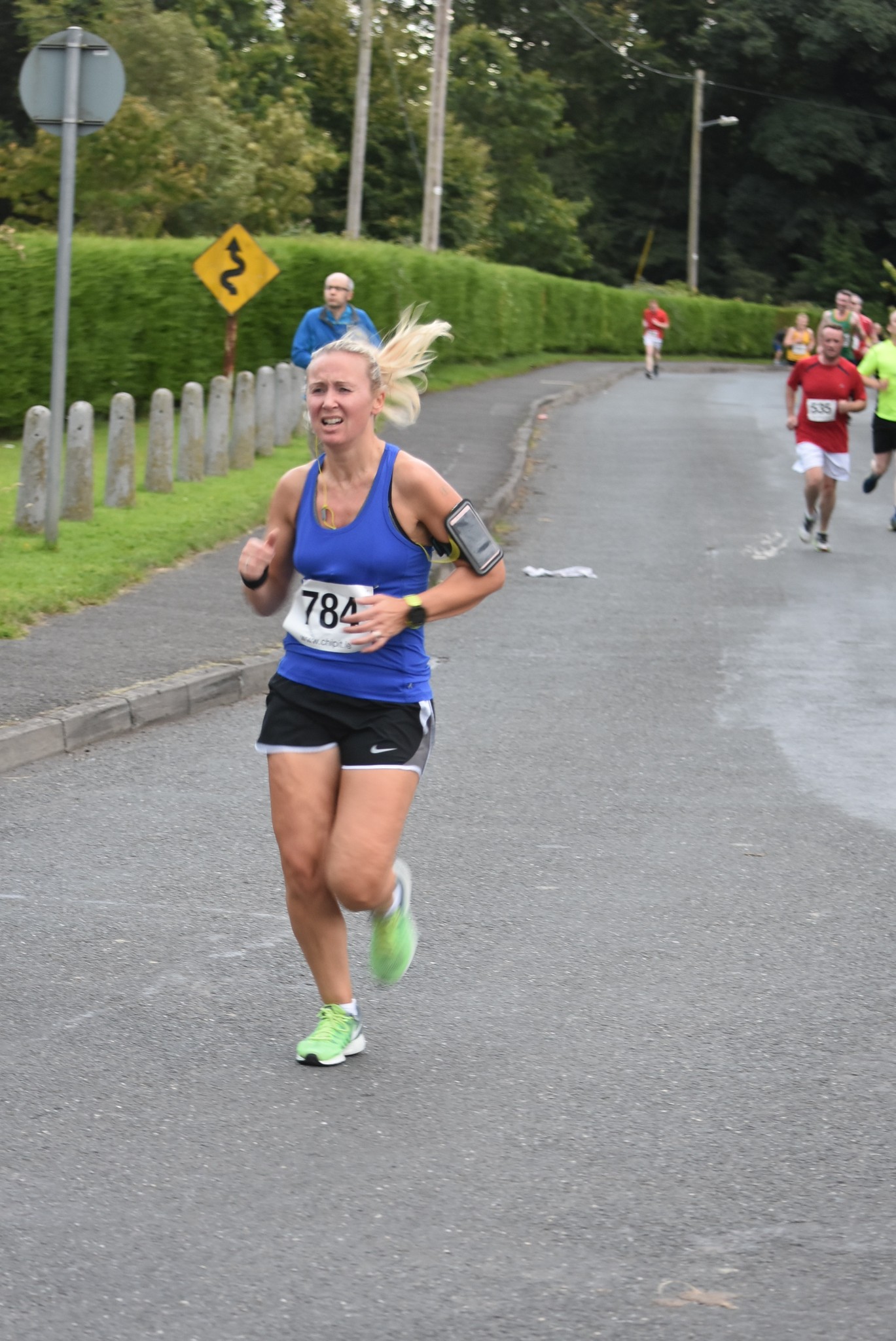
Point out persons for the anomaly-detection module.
[773,290,885,422]
[786,323,867,552]
[237,304,504,1069]
[291,273,383,458]
[642,300,669,380]
[858,307,896,532]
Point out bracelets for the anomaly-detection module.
[240,566,268,590]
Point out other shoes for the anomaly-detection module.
[647,365,659,379]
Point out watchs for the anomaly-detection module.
[403,595,426,629]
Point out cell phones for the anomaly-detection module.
[450,504,500,570]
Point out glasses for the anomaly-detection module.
[324,284,352,293]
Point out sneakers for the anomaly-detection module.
[369,860,417,984]
[889,516,895,533]
[863,474,880,493]
[800,515,816,543]
[293,1005,368,1067]
[816,532,830,551]
